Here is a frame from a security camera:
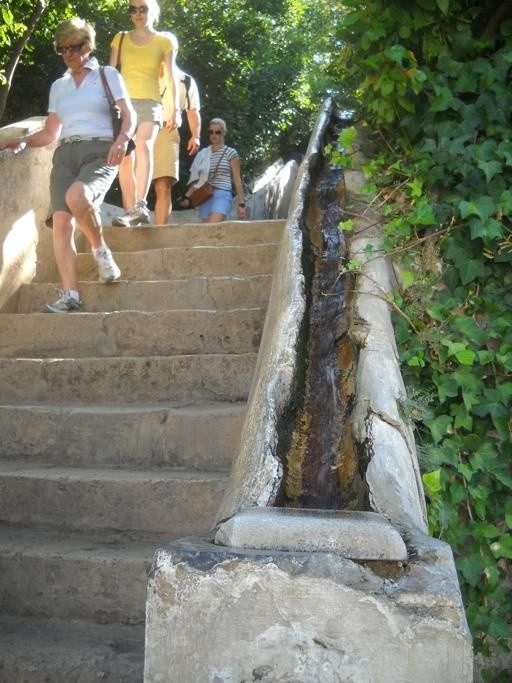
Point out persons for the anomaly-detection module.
[109,0,179,225]
[0,14,139,313]
[149,30,202,224]
[180,116,247,222]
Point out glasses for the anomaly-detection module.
[55,41,86,54]
[126,4,148,15]
[207,129,222,136]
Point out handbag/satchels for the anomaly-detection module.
[99,64,136,157]
[189,181,214,207]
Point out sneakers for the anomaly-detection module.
[41,289,83,314]
[129,202,150,224]
[112,211,141,227]
[91,243,121,284]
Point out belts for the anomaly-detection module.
[58,134,114,144]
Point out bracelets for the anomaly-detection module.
[120,130,132,141]
[238,202,246,207]
[173,106,180,112]
[182,195,189,200]
